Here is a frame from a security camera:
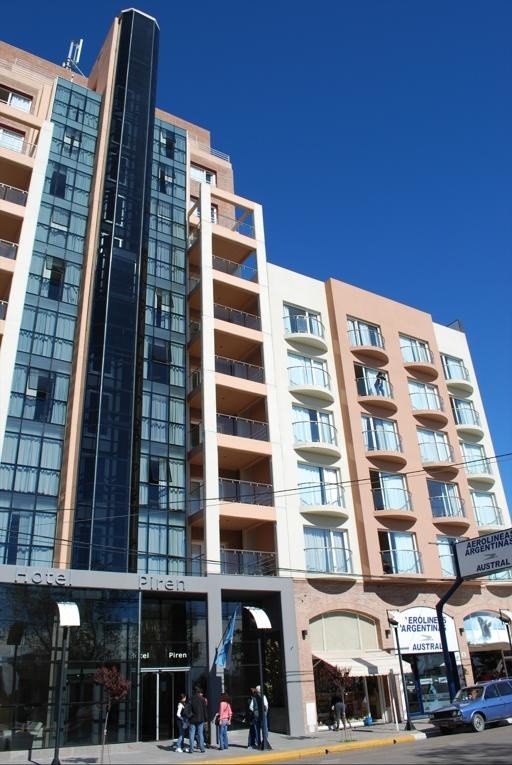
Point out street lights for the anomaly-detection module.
[5,616,26,743]
[45,597,83,765]
[243,602,279,753]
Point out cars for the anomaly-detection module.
[406,674,449,693]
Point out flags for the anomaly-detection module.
[214,611,237,671]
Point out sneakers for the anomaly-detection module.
[217,748,229,751]
[201,751,206,753]
[190,751,195,754]
[182,748,189,752]
[175,748,184,753]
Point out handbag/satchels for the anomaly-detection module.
[214,715,220,726]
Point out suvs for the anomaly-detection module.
[427,678,511,734]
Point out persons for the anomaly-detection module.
[172,684,269,753]
[374,372,386,396]
[331,696,347,731]
[467,693,473,699]
